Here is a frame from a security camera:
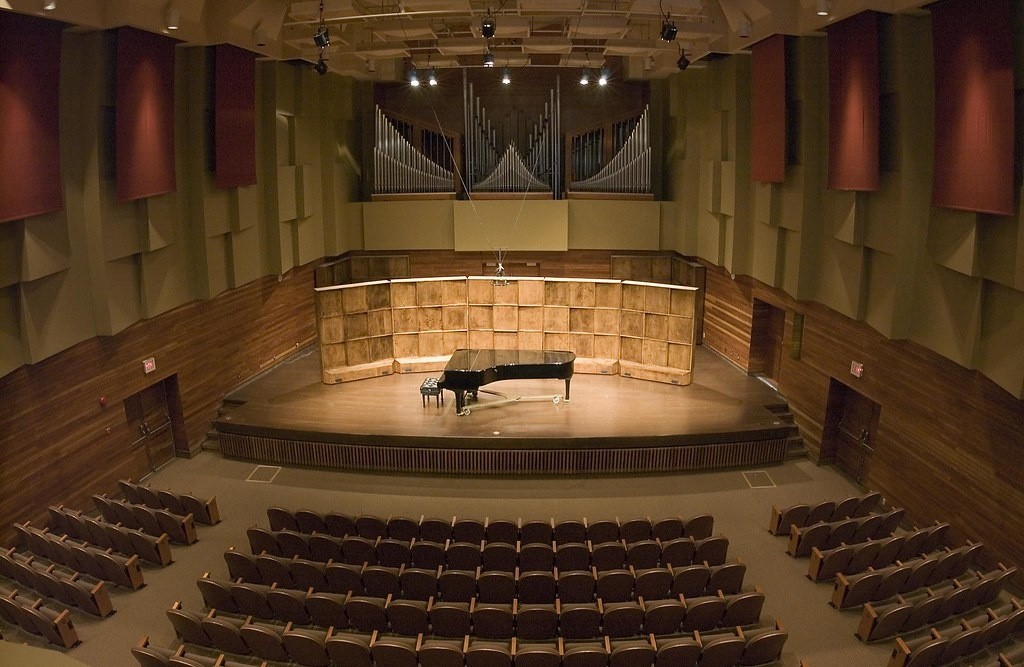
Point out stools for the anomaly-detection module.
[420,377,444,408]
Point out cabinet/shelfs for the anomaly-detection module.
[543,277,621,375]
[467,276,545,349]
[619,279,700,386]
[390,276,468,373]
[314,280,395,384]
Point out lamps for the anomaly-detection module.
[313,0,331,49]
[502,67,511,84]
[410,66,420,87]
[676,48,691,70]
[580,67,589,85]
[740,23,748,37]
[368,60,375,72]
[168,9,179,29]
[44,0,55,10]
[482,8,495,39]
[257,29,266,47]
[599,69,610,85]
[659,12,678,42]
[428,69,438,86]
[817,0,829,16]
[482,45,495,68]
[313,52,330,75]
[644,57,652,70]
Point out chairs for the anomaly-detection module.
[0,480,1024,667]
